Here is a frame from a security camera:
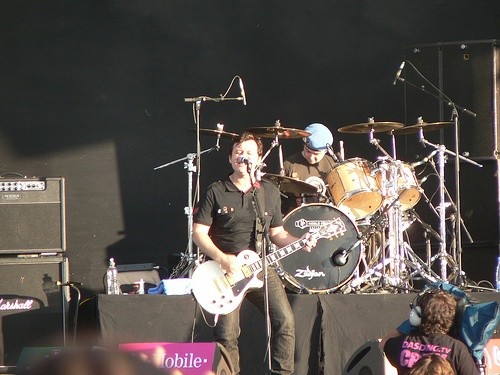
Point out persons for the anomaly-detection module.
[381,289,480,375]
[193,132,317,375]
[283,123,335,206]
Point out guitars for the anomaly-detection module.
[191,216,347,316]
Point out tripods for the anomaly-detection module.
[152,95,239,279]
[321,128,471,294]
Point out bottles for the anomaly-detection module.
[496,257,500,290]
[107,257,120,295]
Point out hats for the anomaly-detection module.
[303,123,334,151]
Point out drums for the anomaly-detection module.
[267,201,365,295]
[326,156,422,232]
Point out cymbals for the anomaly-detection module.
[337,121,456,135]
[243,125,311,139]
[190,128,241,139]
[262,172,318,195]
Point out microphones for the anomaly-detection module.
[393,62,405,86]
[326,145,339,163]
[238,78,247,106]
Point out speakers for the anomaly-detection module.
[15,342,234,375]
[0,177,66,254]
[405,40,500,164]
[404,164,500,249]
[340,338,398,375]
[409,244,500,293]
[0,256,70,373]
